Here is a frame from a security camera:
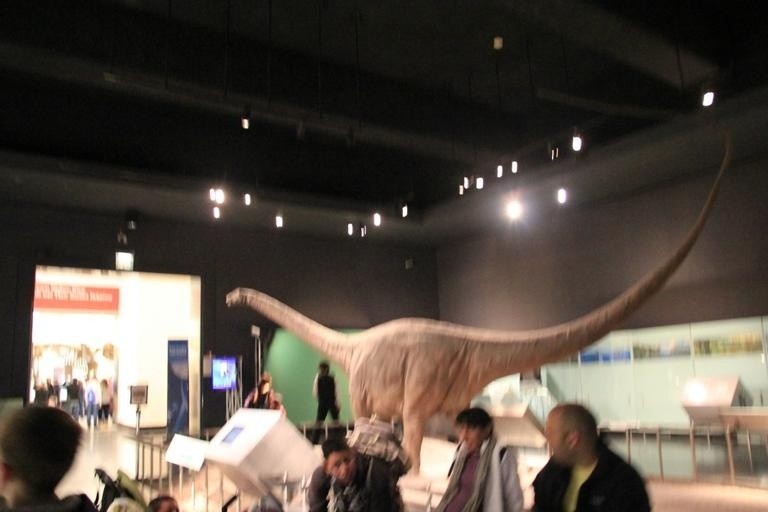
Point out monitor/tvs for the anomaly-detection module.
[211,358,238,390]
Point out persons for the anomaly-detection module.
[1,397,97,512]
[243,380,272,409]
[312,361,341,445]
[34,377,114,429]
[308,436,411,512]
[432,407,524,511]
[147,496,179,511]
[108,497,144,512]
[528,404,651,512]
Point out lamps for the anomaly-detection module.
[125,215,138,231]
[551,143,558,161]
[358,218,368,240]
[238,111,252,130]
